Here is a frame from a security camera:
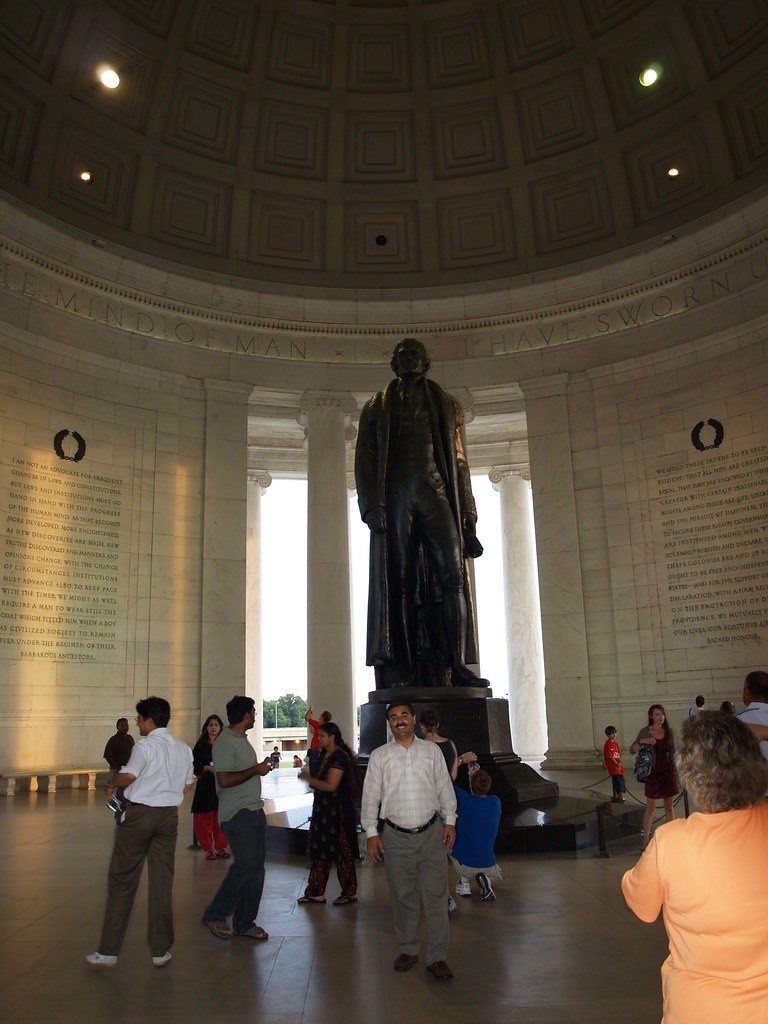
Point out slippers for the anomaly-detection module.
[297,896,326,904]
[333,895,357,905]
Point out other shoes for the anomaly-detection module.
[234,923,268,940]
[206,851,229,859]
[427,959,454,981]
[394,953,419,971]
[200,916,232,939]
[87,953,118,967]
[153,951,173,967]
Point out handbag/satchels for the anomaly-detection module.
[634,752,651,781]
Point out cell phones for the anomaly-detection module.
[310,710,312,714]
[266,762,274,769]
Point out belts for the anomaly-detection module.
[387,811,437,834]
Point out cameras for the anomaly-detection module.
[106,796,123,812]
[469,760,476,767]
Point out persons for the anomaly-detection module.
[103,718,135,825]
[86,696,194,967]
[630,704,679,852]
[732,671,768,762]
[418,708,458,913]
[186,714,231,861]
[687,695,706,718]
[603,726,627,803]
[353,338,491,690]
[621,710,768,1024]
[448,752,502,902]
[296,723,360,904]
[304,705,333,788]
[202,695,275,939]
[720,701,736,716]
[271,746,282,768]
[293,755,302,767]
[361,701,458,982]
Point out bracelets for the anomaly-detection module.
[459,756,464,764]
[106,783,114,789]
[637,739,642,747]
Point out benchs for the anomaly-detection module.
[0,768,110,797]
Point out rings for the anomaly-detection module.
[652,741,653,743]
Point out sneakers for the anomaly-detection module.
[448,897,456,912]
[476,872,496,902]
[455,879,472,896]
